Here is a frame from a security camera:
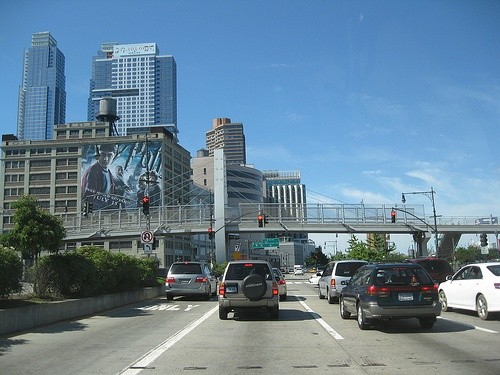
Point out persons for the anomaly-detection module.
[82,143,118,213]
[137,171,161,207]
[113,165,131,206]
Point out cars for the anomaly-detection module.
[308,271,323,285]
[340,262,441,330]
[438,262,500,320]
[271,267,287,301]
[293,264,304,276]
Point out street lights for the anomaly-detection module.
[402,187,439,258]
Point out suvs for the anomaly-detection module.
[316,260,368,305]
[403,257,455,284]
[218,260,279,321]
[164,261,218,301]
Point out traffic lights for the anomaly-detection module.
[208,228,213,240]
[391,211,395,223]
[258,215,263,227]
[143,196,150,214]
[263,214,268,227]
[480,233,488,246]
[82,201,93,216]
